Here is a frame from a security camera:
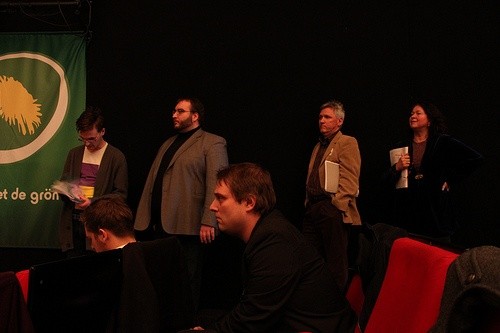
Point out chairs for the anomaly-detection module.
[0,234,199,333]
[344,222,461,333]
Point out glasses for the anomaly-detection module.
[173,109,196,115]
[78,133,99,144]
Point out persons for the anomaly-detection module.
[383,98,462,238]
[79,194,140,253]
[58,108,128,249]
[179,162,343,333]
[303,101,362,284]
[134,97,229,316]
